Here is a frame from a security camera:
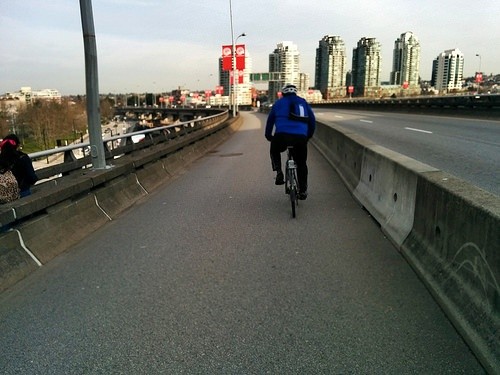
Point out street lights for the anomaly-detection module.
[230,31,248,116]
[475,53,481,93]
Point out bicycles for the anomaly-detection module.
[284,146,299,218]
